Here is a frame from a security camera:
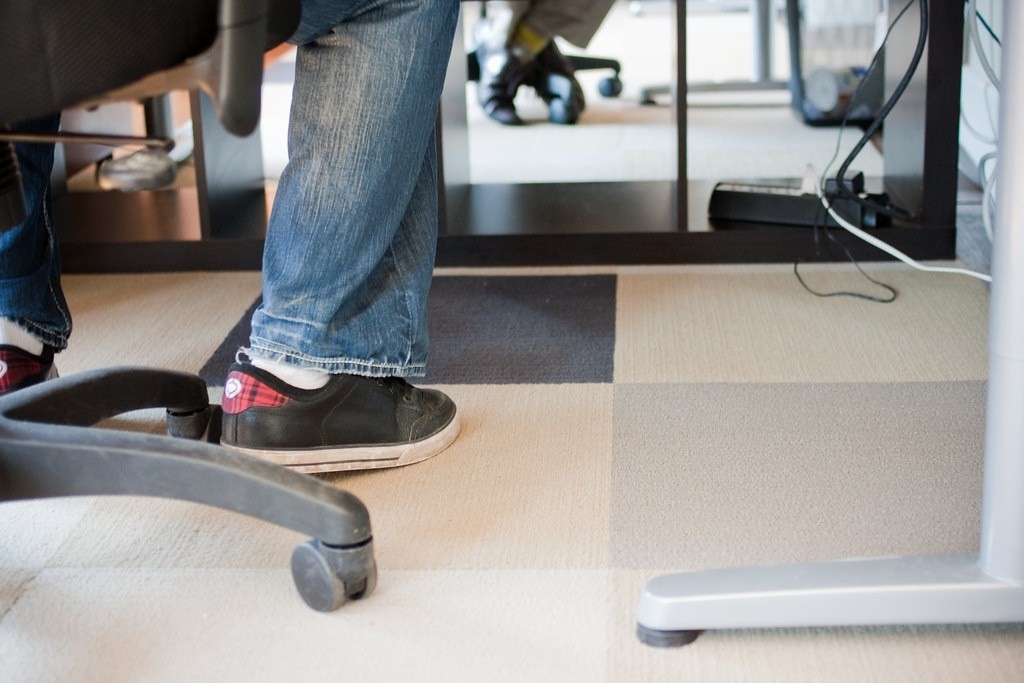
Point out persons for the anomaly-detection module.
[471,1,617,126]
[0,0,464,478]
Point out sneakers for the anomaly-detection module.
[0,316,61,397]
[220,363,459,474]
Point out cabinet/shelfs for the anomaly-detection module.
[45,0,965,274]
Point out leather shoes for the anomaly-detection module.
[539,66,585,125]
[471,15,523,126]
